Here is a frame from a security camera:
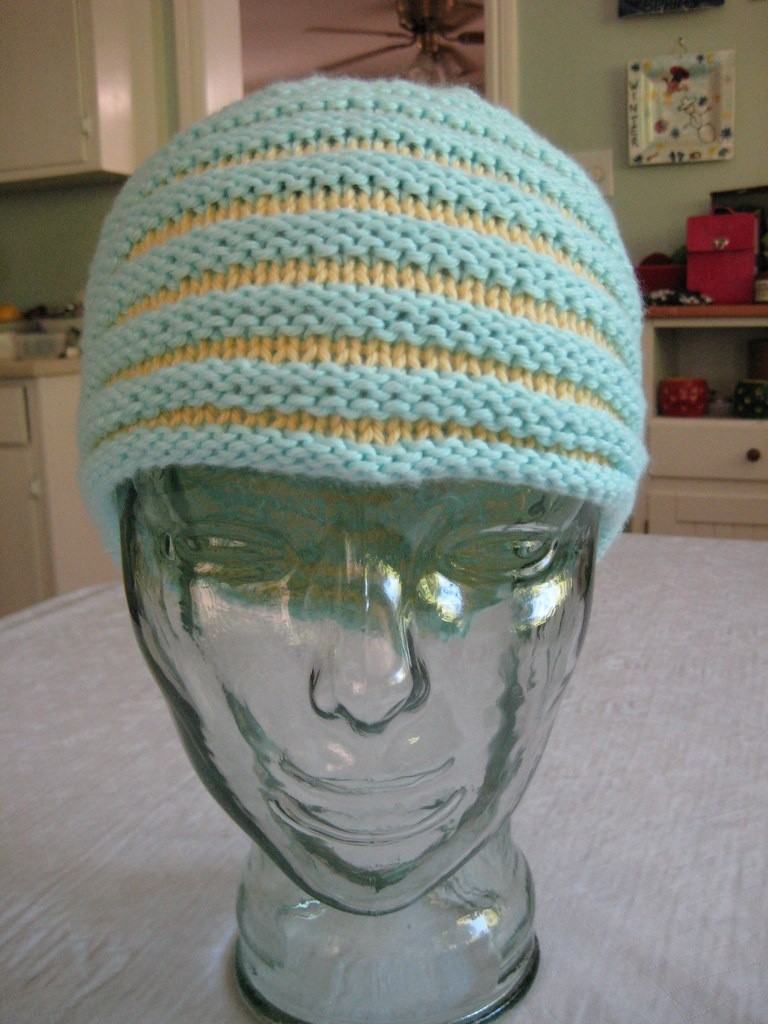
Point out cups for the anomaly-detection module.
[659,377,708,418]
[735,378,768,418]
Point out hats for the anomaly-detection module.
[74,74,648,565]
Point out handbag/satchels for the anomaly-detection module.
[635,205,759,305]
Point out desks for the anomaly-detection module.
[1,534,768,1023]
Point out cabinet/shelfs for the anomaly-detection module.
[0,377,122,614]
[643,305,768,540]
[1,0,162,192]
